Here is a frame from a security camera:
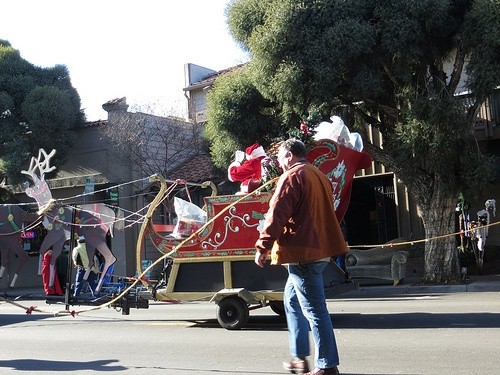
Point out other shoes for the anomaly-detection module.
[283,361,308,374]
[304,367,339,375]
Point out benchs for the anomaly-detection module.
[258,156,283,193]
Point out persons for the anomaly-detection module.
[254,139,349,375]
[227,143,269,195]
[42,236,114,303]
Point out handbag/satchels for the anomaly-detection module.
[171,196,208,240]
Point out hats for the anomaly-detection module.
[245,144,266,160]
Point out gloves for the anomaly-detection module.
[236,149,246,164]
